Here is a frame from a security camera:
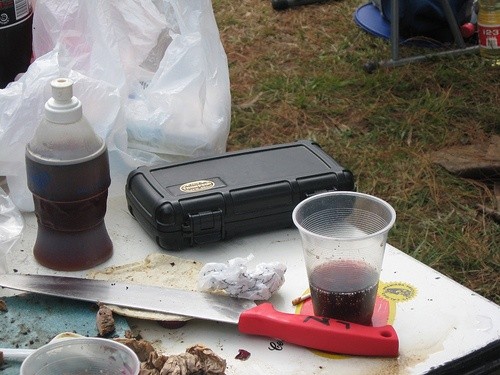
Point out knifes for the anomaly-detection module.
[0,273,400,357]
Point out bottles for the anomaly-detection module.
[25,77,113,270]
[477,0,500,66]
[0,0,34,88]
[461,0,479,36]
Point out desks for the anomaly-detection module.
[1,176,500,375]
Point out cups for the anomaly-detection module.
[292,190,397,325]
[18,336,142,375]
